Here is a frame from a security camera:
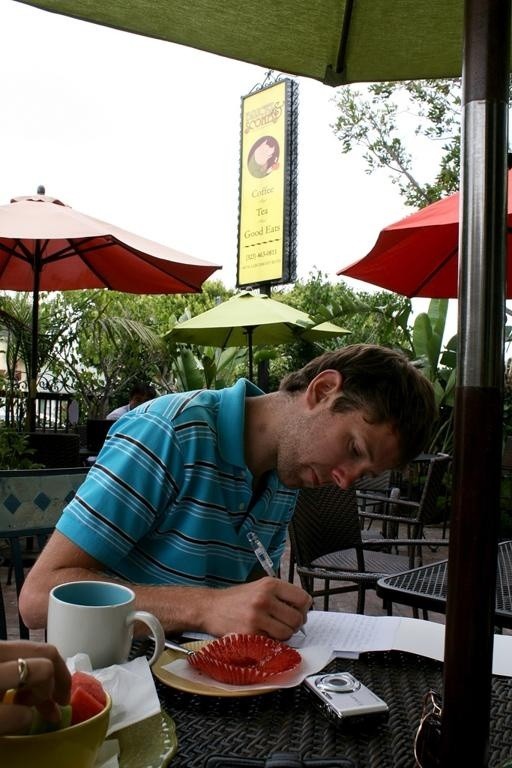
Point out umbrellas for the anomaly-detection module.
[0,186,225,432]
[337,166,511,300]
[17,0,512,767]
[162,286,353,388]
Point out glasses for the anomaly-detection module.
[416,689,442,768]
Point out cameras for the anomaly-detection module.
[305,671,391,732]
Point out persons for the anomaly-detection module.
[84,383,156,466]
[20,342,436,640]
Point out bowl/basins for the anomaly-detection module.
[1,686,110,768]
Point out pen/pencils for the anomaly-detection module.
[248,533,309,639]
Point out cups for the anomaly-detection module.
[46,578,163,672]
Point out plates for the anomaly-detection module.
[108,707,180,768]
[154,639,275,693]
